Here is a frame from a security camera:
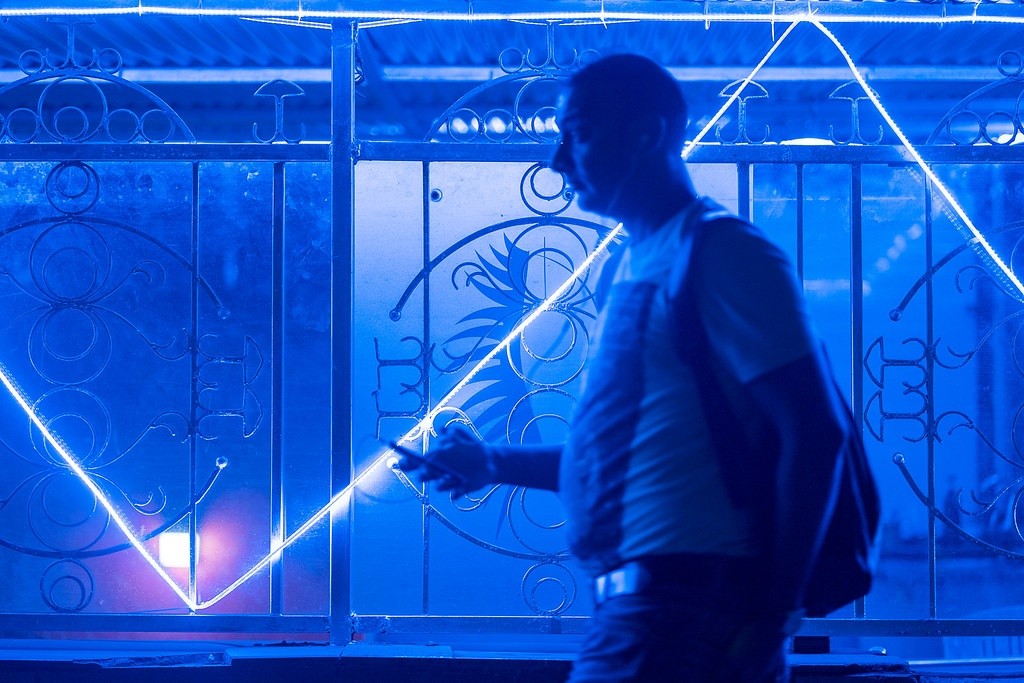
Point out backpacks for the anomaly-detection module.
[593,210,879,616]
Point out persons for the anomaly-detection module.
[390,50,881,683]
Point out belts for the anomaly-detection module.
[586,564,661,603]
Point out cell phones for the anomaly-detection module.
[389,440,471,488]
